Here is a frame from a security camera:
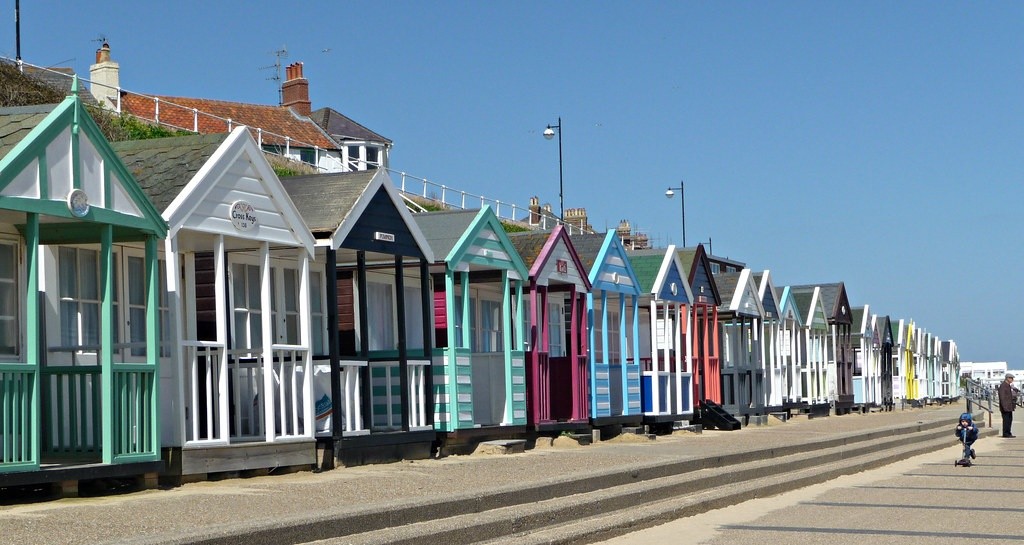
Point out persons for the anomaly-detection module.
[956,413,978,463]
[974,378,990,400]
[998,373,1018,437]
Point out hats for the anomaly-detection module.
[1006,374,1015,378]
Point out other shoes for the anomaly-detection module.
[964,457,969,463]
[1003,435,1016,438]
[971,449,976,459]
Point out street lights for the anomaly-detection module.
[543,116,563,222]
[665,180,685,247]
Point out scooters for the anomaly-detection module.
[954,427,972,468]
